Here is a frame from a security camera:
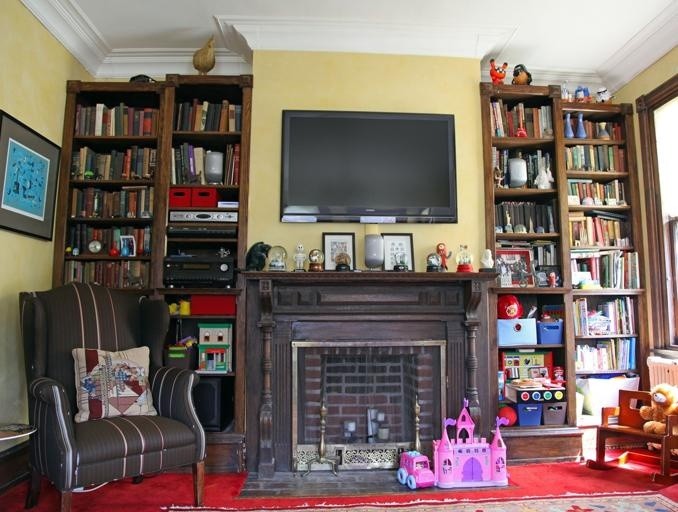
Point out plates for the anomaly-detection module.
[1,422,38,441]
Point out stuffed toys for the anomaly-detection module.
[553,366,564,387]
[561,79,614,104]
[498,406,517,427]
[511,63,532,85]
[489,58,508,85]
[639,382,678,435]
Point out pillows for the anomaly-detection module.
[69,344,159,423]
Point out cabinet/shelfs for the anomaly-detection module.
[561,102,652,434]
[478,82,585,465]
[160,72,253,475]
[52,79,166,295]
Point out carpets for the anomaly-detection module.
[163,491,678,512]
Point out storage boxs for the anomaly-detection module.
[514,402,544,429]
[169,187,192,207]
[190,294,237,315]
[538,319,564,345]
[544,401,570,426]
[577,373,644,421]
[192,187,220,209]
[497,318,540,349]
[187,378,233,432]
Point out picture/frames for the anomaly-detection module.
[380,231,417,273]
[119,233,137,258]
[495,247,536,287]
[320,232,357,274]
[0,108,64,242]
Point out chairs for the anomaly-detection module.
[20,282,207,512]
[595,390,678,488]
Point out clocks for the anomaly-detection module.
[87,238,104,255]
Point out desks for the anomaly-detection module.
[0,421,38,443]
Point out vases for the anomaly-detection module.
[564,109,612,141]
[110,239,119,257]
[121,238,130,257]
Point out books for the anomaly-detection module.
[169,97,242,186]
[63,100,158,289]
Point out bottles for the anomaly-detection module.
[576,112,587,139]
[597,122,610,141]
[563,113,575,138]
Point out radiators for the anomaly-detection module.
[645,355,678,396]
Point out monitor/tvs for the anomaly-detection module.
[281,109,457,223]
